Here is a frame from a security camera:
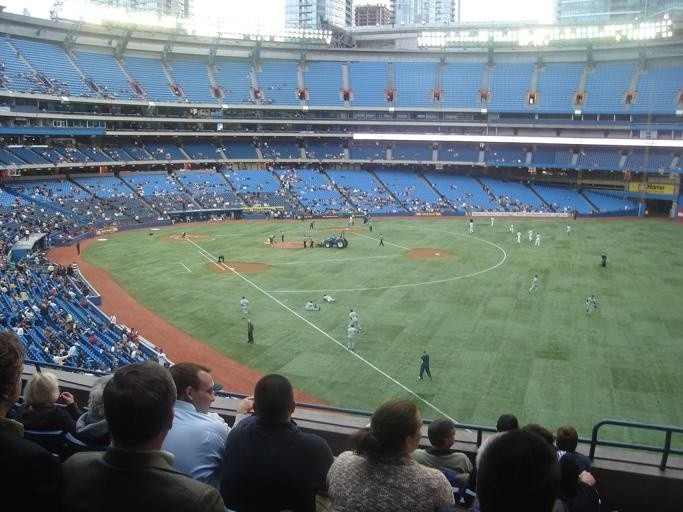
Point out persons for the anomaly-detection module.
[0,64,681,512]
